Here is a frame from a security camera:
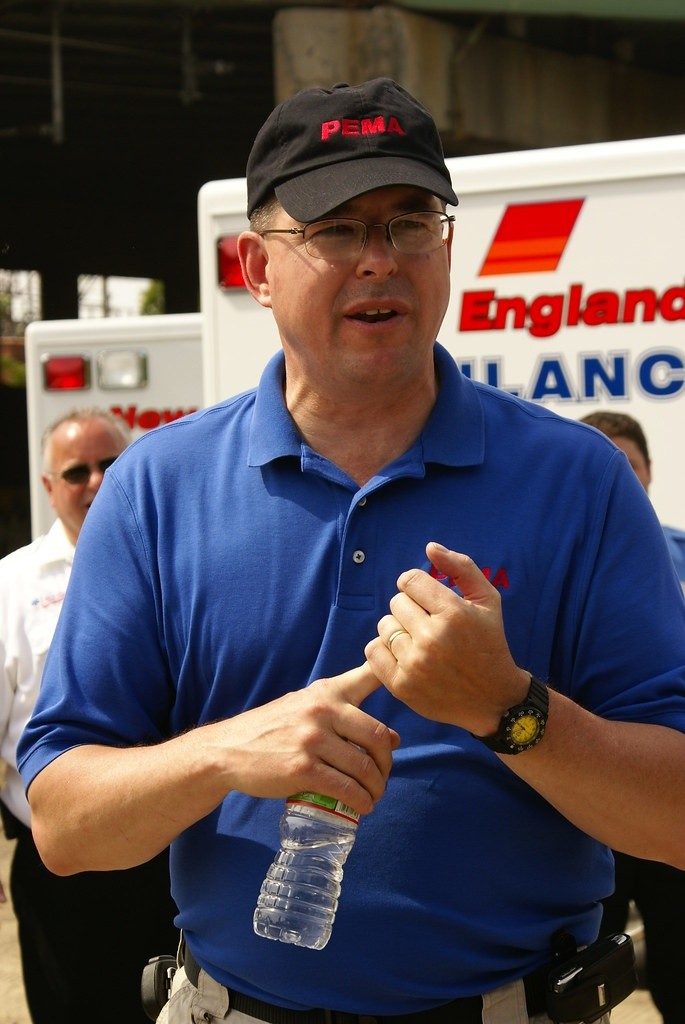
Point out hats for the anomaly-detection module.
[246,77,459,222]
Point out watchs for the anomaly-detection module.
[471,670,549,754]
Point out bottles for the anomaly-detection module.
[251,790,361,950]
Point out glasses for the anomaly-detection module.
[49,457,124,485]
[253,211,455,260]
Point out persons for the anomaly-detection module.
[17,75,685,1024]
[1,409,181,1023]
[577,411,685,1024]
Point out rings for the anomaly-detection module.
[386,629,408,653]
